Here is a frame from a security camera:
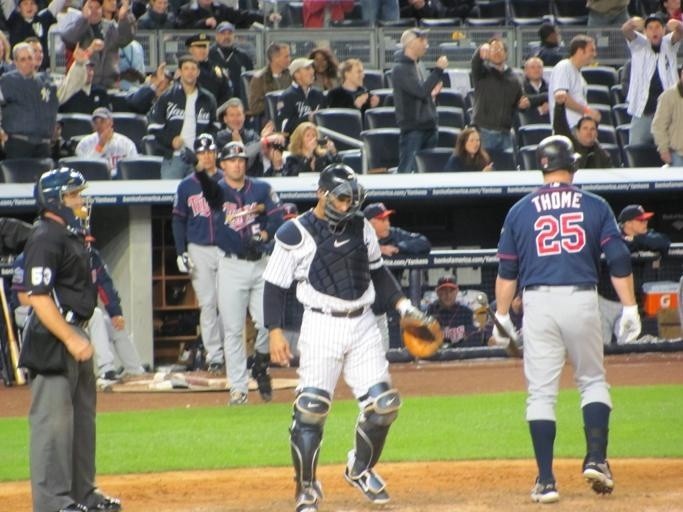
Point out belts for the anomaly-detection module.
[310,305,363,318]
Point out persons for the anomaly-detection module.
[2,216,130,394]
[173,132,673,401]
[1,0,683,179]
[263,159,441,512]
[491,133,643,503]
[17,166,129,512]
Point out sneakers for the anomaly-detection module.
[208,363,224,376]
[296,493,317,511]
[580,455,614,494]
[227,392,248,406]
[531,476,559,502]
[254,368,273,396]
[346,465,388,505]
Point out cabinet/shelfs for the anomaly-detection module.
[151,214,201,345]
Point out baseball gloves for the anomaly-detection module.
[398,301,444,359]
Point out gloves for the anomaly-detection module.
[492,312,517,345]
[617,305,642,342]
[397,298,420,318]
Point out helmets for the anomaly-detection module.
[537,135,581,173]
[38,165,86,211]
[319,163,367,236]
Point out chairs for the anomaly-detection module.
[0,0,683,183]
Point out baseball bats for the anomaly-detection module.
[3,277,31,387]
[474,294,522,357]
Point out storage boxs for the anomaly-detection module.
[641,280,680,317]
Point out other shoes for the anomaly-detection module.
[58,504,88,512]
[101,366,126,380]
[93,497,121,512]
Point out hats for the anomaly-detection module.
[400,28,430,47]
[363,202,395,220]
[436,275,458,293]
[619,205,653,222]
[92,21,314,161]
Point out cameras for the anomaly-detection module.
[316,137,327,144]
